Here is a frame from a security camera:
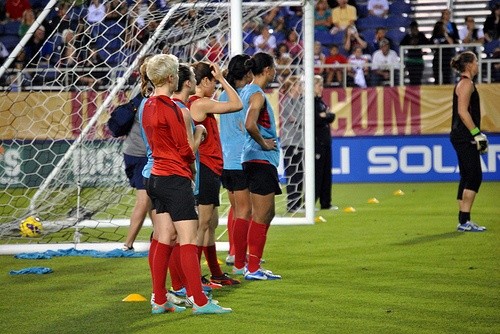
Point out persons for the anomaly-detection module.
[236,52,282,281]
[311,75,339,213]
[449,52,488,228]
[220,55,266,266]
[170,62,224,299]
[0,1,499,88]
[190,63,240,289]
[122,84,158,252]
[281,74,306,214]
[141,54,232,314]
[140,57,220,307]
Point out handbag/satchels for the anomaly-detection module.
[107,89,145,137]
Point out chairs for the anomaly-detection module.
[0,0,414,85]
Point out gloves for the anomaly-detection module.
[471,126,490,154]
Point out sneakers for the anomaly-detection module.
[457,220,488,232]
[122,242,281,314]
